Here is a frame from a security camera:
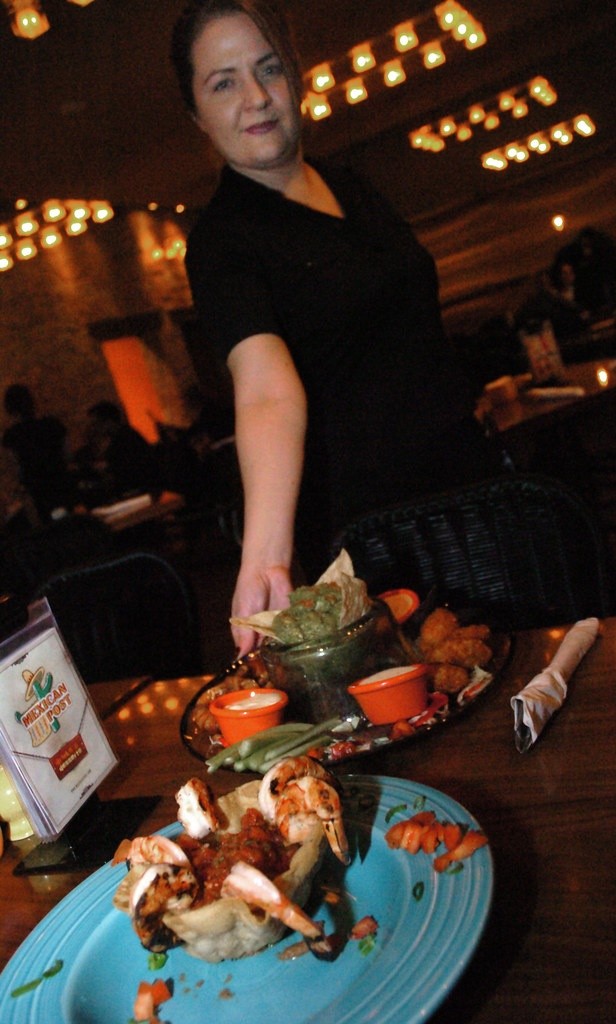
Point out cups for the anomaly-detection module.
[269,597,418,724]
[349,663,429,724]
[211,689,288,749]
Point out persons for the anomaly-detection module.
[0,382,235,640]
[452,227,616,386]
[169,0,503,651]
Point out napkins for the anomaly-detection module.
[510,618,600,743]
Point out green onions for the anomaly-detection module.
[149,953,166,969]
[445,862,463,875]
[413,882,424,900]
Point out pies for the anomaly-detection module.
[228,549,370,645]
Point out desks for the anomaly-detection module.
[474,355,616,492]
[0,614,616,1024]
[89,496,184,534]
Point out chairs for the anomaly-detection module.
[179,447,243,574]
[0,516,122,594]
[323,471,616,628]
[12,547,206,678]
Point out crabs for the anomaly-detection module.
[114,755,352,954]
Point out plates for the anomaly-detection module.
[178,631,511,774]
[0,776,496,1024]
